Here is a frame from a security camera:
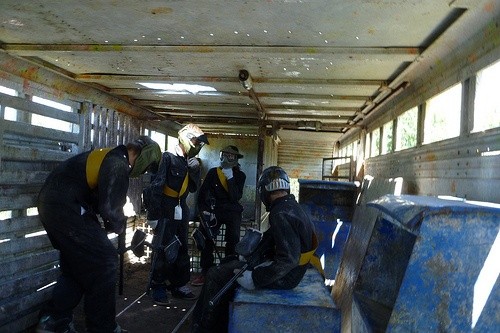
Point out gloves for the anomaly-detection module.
[222,167,233,180]
[189,158,200,168]
[203,211,216,221]
[147,220,158,230]
[233,268,255,291]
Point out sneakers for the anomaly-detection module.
[149,286,171,305]
[85,320,128,333]
[36,311,78,333]
[171,287,197,299]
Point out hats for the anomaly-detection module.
[220,145,243,159]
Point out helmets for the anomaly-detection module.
[258,166,290,194]
[128,135,161,178]
[178,123,209,157]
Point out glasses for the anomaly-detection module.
[220,152,237,162]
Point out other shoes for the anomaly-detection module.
[191,275,206,286]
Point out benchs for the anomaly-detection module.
[223,266,340,333]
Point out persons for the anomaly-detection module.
[37,137,161,333]
[194,166,319,333]
[192,145,246,285]
[148,123,209,305]
